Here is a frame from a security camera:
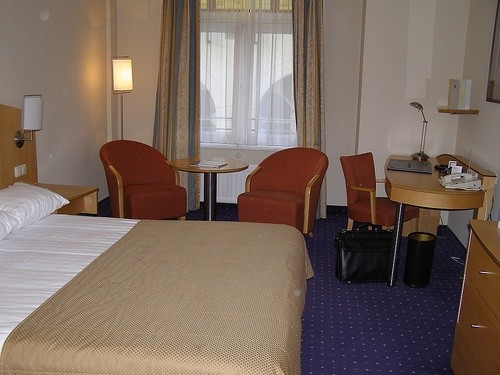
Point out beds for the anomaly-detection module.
[0,104,315,375]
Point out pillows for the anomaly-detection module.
[0,181,70,241]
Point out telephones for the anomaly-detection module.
[440,173,482,190]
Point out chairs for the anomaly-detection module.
[339,152,420,233]
[100,140,187,220]
[238,148,329,234]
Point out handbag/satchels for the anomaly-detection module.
[335,222,392,283]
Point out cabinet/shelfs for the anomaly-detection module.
[442,219,500,375]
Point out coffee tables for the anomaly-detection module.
[170,156,249,220]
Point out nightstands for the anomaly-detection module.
[33,182,99,216]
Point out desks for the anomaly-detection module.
[384,154,497,287]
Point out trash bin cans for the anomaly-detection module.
[403,231,437,288]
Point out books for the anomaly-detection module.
[190,160,227,168]
[448,79,472,109]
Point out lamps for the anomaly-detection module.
[112,56,134,140]
[410,102,431,161]
[14,95,44,148]
